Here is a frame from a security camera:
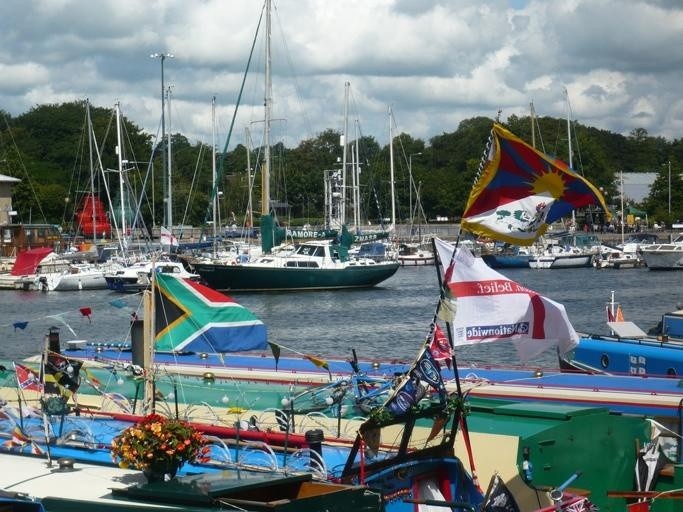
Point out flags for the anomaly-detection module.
[485,475,520,512]
[459,123,613,246]
[434,235,579,365]
[152,273,268,353]
[13,359,44,392]
[40,348,84,404]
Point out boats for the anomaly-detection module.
[556,289,682,379]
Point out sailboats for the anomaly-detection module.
[1,1,682,292]
